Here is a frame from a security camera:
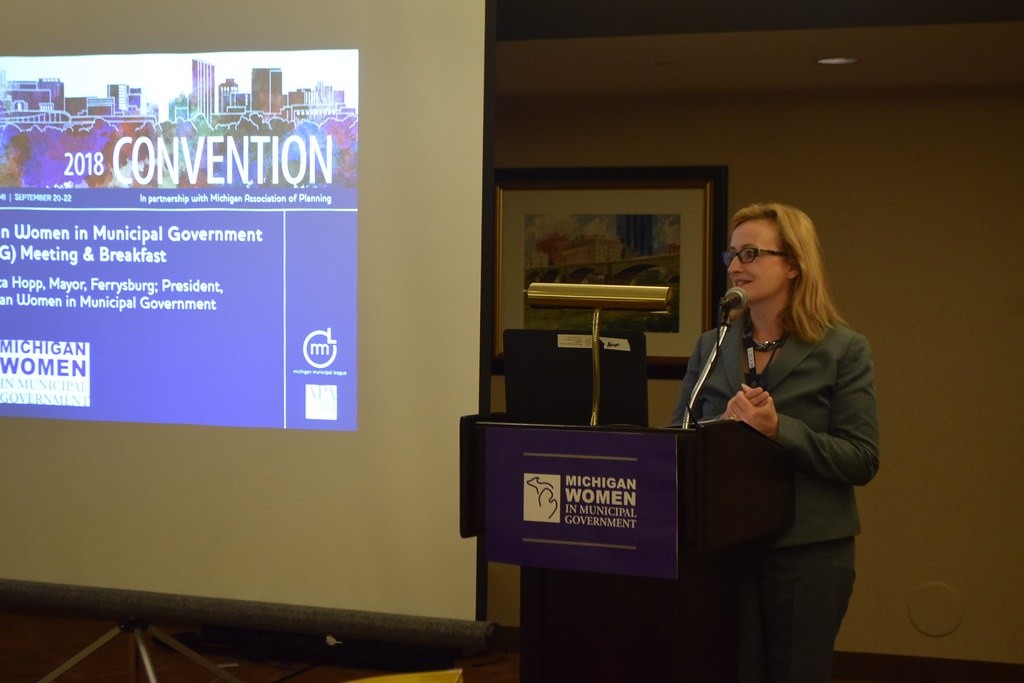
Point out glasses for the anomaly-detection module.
[721,248,793,266]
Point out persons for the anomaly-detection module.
[670,202,880,683]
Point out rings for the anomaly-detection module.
[729,414,737,420]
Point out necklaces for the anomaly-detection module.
[742,319,787,352]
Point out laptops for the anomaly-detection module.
[502,328,650,428]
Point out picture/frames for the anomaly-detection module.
[489,164,728,381]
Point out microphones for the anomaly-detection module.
[718,286,747,311]
